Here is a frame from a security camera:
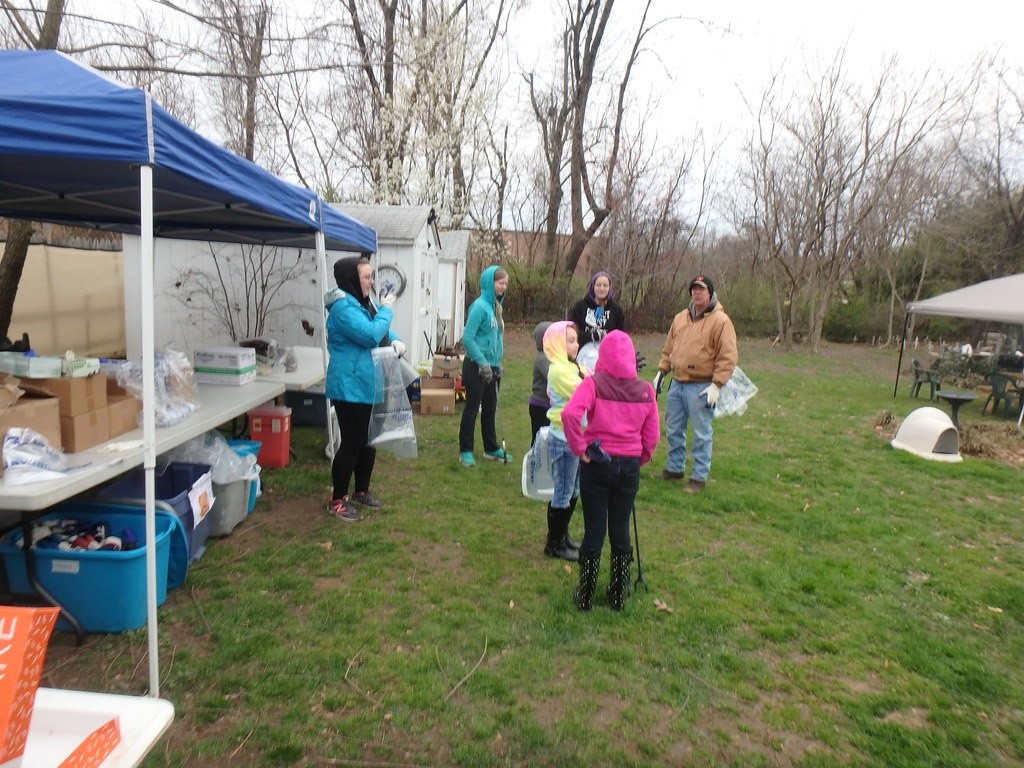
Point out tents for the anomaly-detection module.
[0,48,378,701]
[894,274,1024,426]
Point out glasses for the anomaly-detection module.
[359,275,375,281]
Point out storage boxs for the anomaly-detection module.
[101,462,212,560]
[0,386,64,477]
[247,406,294,468]
[411,376,420,403]
[63,353,102,378]
[0,349,62,379]
[432,353,460,378]
[211,472,254,539]
[194,346,256,385]
[0,369,107,417]
[63,405,110,450]
[418,376,456,415]
[109,394,139,438]
[0,513,177,637]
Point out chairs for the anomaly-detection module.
[908,357,943,403]
[980,371,1020,420]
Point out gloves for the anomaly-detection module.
[636,350,647,370]
[479,365,492,383]
[495,365,504,380]
[700,383,720,409]
[381,288,397,307]
[653,370,665,393]
[390,340,406,357]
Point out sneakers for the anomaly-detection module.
[326,495,363,521]
[482,448,512,462]
[351,491,384,510]
[459,451,475,467]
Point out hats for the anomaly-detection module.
[689,275,714,299]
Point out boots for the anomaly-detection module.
[573,549,601,610]
[607,546,634,610]
[565,496,582,549]
[544,502,579,561]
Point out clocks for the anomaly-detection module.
[372,263,407,299]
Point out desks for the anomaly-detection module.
[21,687,176,768]
[934,391,978,425]
[253,346,330,459]
[1009,387,1024,415]
[0,383,287,697]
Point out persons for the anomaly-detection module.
[543,321,647,561]
[529,321,551,486]
[650,274,738,495]
[459,265,513,468]
[568,272,624,353]
[561,329,661,611]
[324,251,406,523]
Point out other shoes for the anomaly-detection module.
[650,469,684,480]
[684,479,705,493]
